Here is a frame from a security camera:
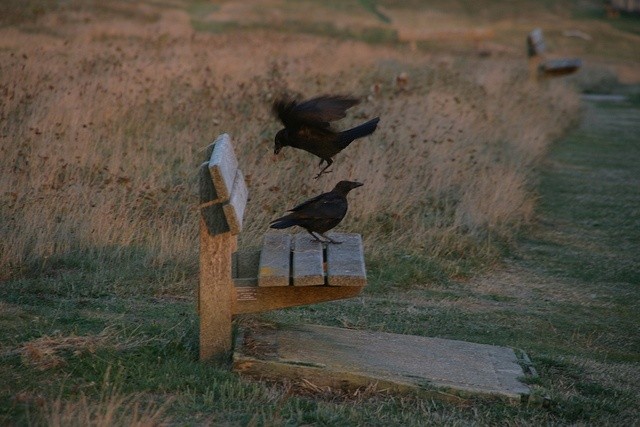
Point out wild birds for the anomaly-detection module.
[268,181,364,245]
[271,95,381,179]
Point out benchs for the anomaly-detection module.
[194,131,367,355]
[527,28,580,79]
